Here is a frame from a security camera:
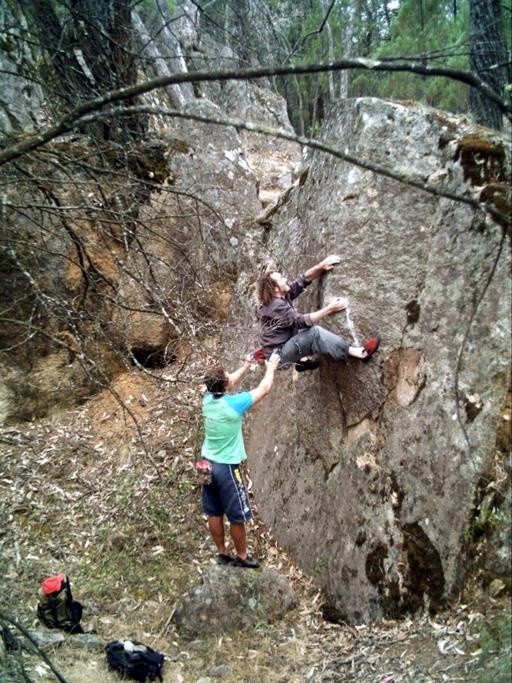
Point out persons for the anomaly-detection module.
[255,254,383,371]
[199,349,282,568]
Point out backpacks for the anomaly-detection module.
[37,575,83,634]
[105,639,164,681]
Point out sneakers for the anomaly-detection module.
[233,556,260,567]
[294,360,323,371]
[216,553,233,565]
[360,338,379,363]
